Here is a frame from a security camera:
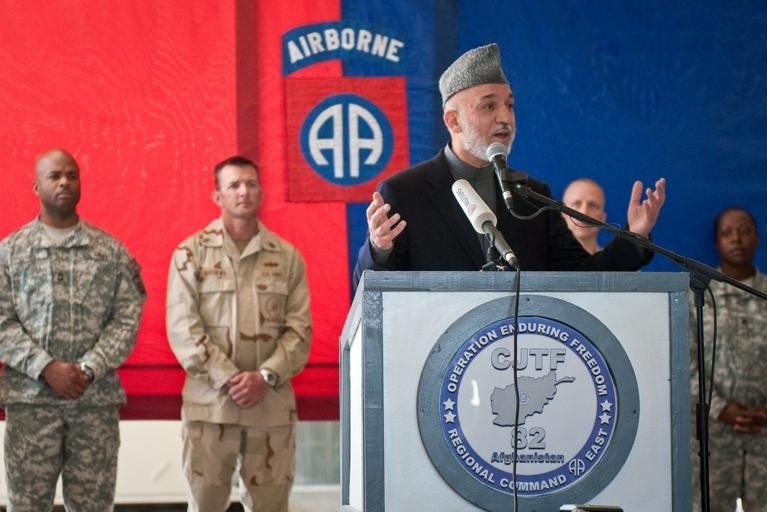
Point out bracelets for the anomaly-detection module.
[79,363,94,381]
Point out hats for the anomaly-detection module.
[438,43,511,103]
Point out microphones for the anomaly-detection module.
[451,178,519,267]
[486,142,516,210]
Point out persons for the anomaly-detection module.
[558,177,607,258]
[685,205,766,512]
[162,157,315,511]
[0,146,147,511]
[350,39,667,278]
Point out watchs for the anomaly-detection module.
[259,368,277,387]
